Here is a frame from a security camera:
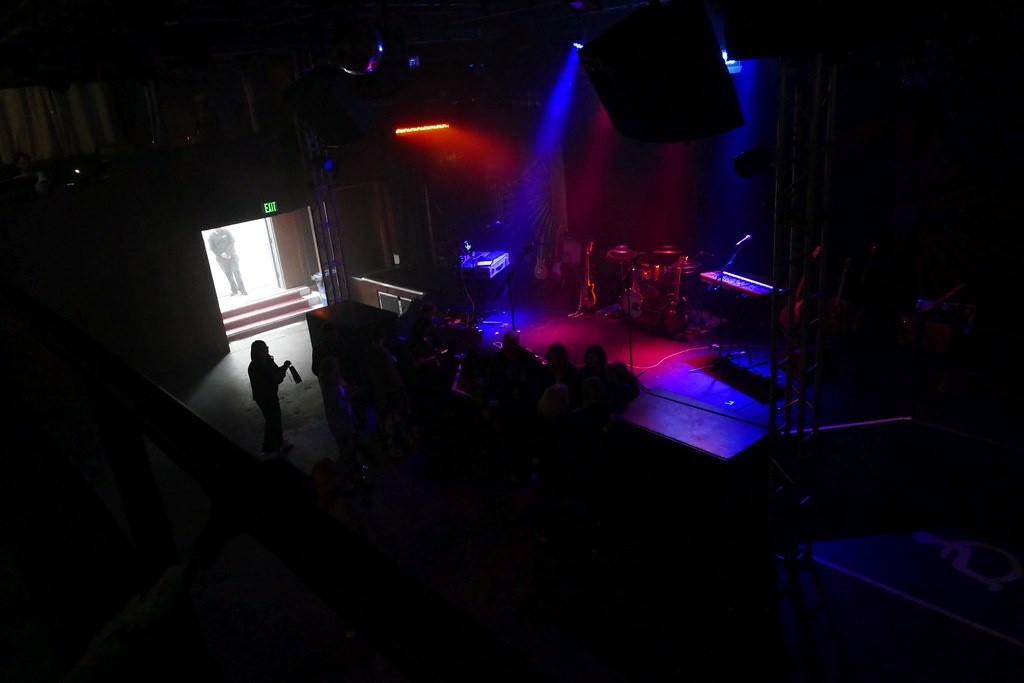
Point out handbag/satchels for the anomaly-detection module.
[289,364,302,384]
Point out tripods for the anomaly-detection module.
[708,253,754,366]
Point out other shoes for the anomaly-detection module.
[239,288,248,295]
[230,290,237,296]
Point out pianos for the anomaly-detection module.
[699,271,775,365]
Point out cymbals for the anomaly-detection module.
[606,244,683,266]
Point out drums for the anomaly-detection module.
[619,282,684,336]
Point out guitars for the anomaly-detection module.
[534,223,599,310]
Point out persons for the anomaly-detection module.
[12,154,38,178]
[309,456,343,516]
[313,303,631,450]
[247,341,294,454]
[189,97,218,145]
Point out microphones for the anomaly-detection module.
[524,242,534,252]
[736,234,752,246]
[631,252,644,262]
